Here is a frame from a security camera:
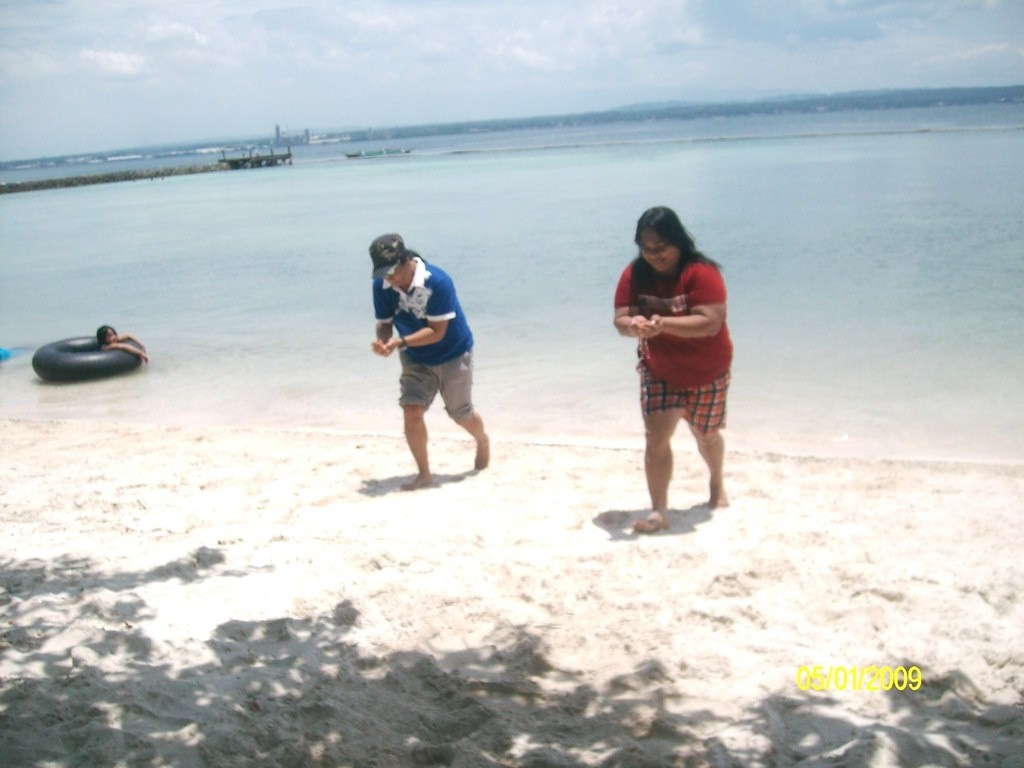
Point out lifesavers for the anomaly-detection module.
[32,335,147,382]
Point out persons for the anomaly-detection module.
[613,206,734,533]
[96,326,149,364]
[369,233,490,491]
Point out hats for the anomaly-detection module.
[369,233,405,278]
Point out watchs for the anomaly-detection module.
[398,338,407,351]
